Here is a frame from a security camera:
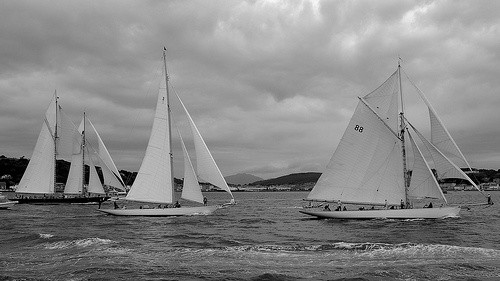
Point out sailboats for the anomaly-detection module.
[8,89,128,204]
[96,46,238,216]
[299,57,495,219]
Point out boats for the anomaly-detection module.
[0,192,19,209]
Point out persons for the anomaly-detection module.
[385,200,390,210]
[69,182,92,199]
[104,185,131,198]
[15,193,66,199]
[203,196,208,206]
[337,199,342,211]
[401,199,404,209]
[486,193,492,204]
[114,201,119,209]
[98,194,102,209]
[158,200,181,208]
[406,198,411,209]
[427,202,433,208]
